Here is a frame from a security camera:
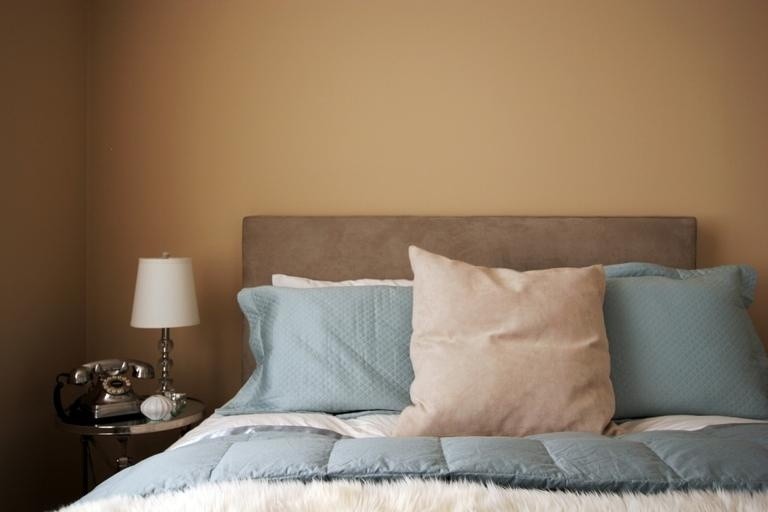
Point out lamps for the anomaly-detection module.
[130,253,200,417]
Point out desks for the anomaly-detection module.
[55,398,205,495]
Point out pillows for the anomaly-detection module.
[271,273,413,291]
[394,244,617,435]
[602,263,767,426]
[214,285,416,416]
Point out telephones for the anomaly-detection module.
[70,359,154,420]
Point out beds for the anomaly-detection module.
[50,215,768,512]
